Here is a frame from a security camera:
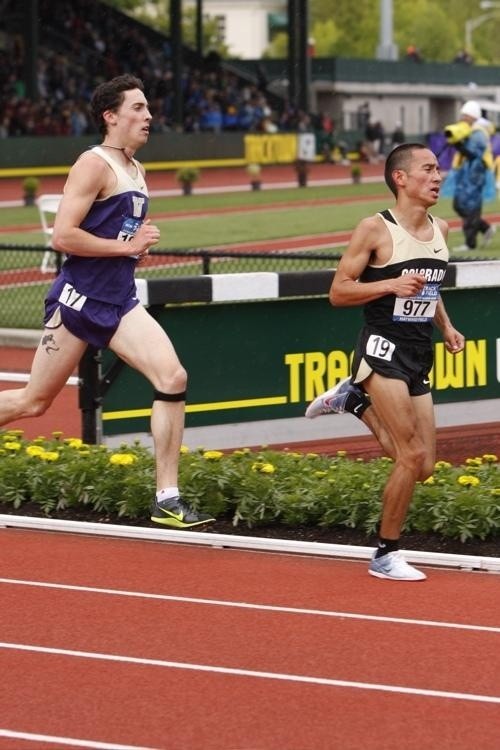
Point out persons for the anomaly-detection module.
[442,101,497,254]
[302,142,469,583]
[1,69,220,532]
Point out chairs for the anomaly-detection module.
[34,194,69,274]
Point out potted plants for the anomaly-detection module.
[294,157,308,187]
[351,166,361,184]
[21,176,39,207]
[246,162,262,192]
[175,166,198,195]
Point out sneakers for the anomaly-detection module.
[480,224,495,248]
[304,375,364,421]
[367,548,427,582]
[450,243,471,254]
[150,495,217,529]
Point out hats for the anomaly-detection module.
[460,99,482,122]
